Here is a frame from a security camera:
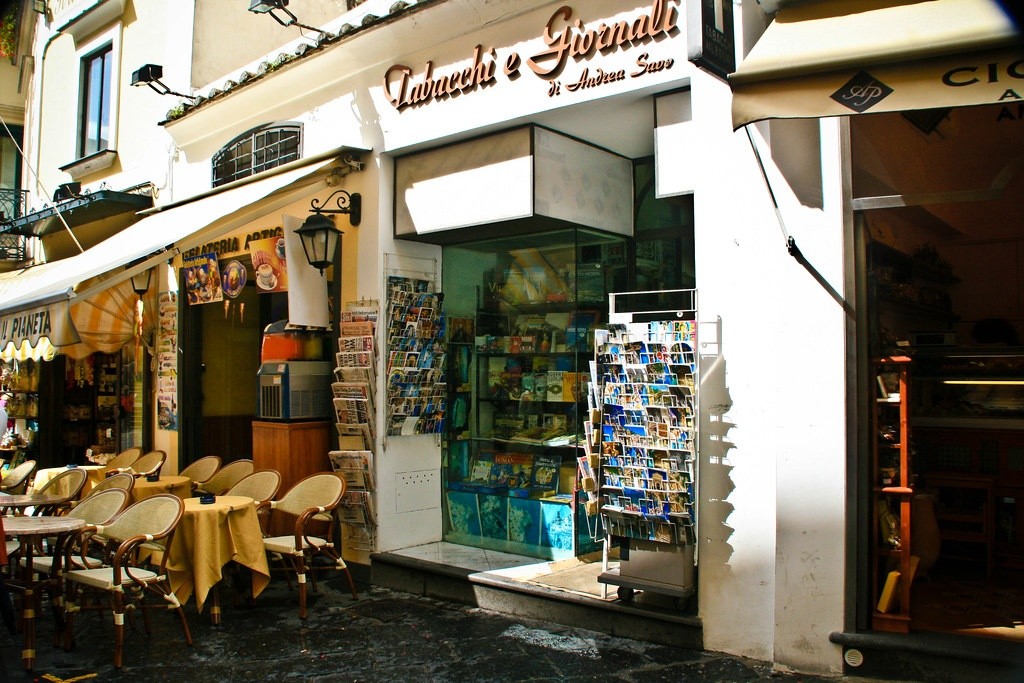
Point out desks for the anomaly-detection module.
[0,516,85,669]
[153,495,272,627]
[29,466,106,518]
[128,476,192,507]
[0,495,68,577]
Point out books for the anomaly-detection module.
[451,452,561,497]
[577,320,697,544]
[387,276,447,436]
[488,371,590,402]
[491,414,580,446]
[510,313,573,352]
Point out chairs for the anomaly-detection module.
[0,445,358,669]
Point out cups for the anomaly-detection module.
[256,264,273,285]
[276,238,285,255]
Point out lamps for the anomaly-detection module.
[126,241,175,301]
[293,190,362,276]
[52,182,91,203]
[128,64,197,99]
[247,0,325,34]
[0,210,8,226]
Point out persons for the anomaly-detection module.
[487,337,497,353]
[509,337,523,353]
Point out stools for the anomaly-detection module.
[918,470,995,588]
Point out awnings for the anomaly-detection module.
[0,156,347,315]
[0,266,159,363]
[727,0,1024,317]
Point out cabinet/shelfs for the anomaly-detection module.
[875,355,913,635]
[472,298,602,493]
[0,390,38,420]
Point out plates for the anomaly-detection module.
[257,275,277,290]
[275,248,285,259]
